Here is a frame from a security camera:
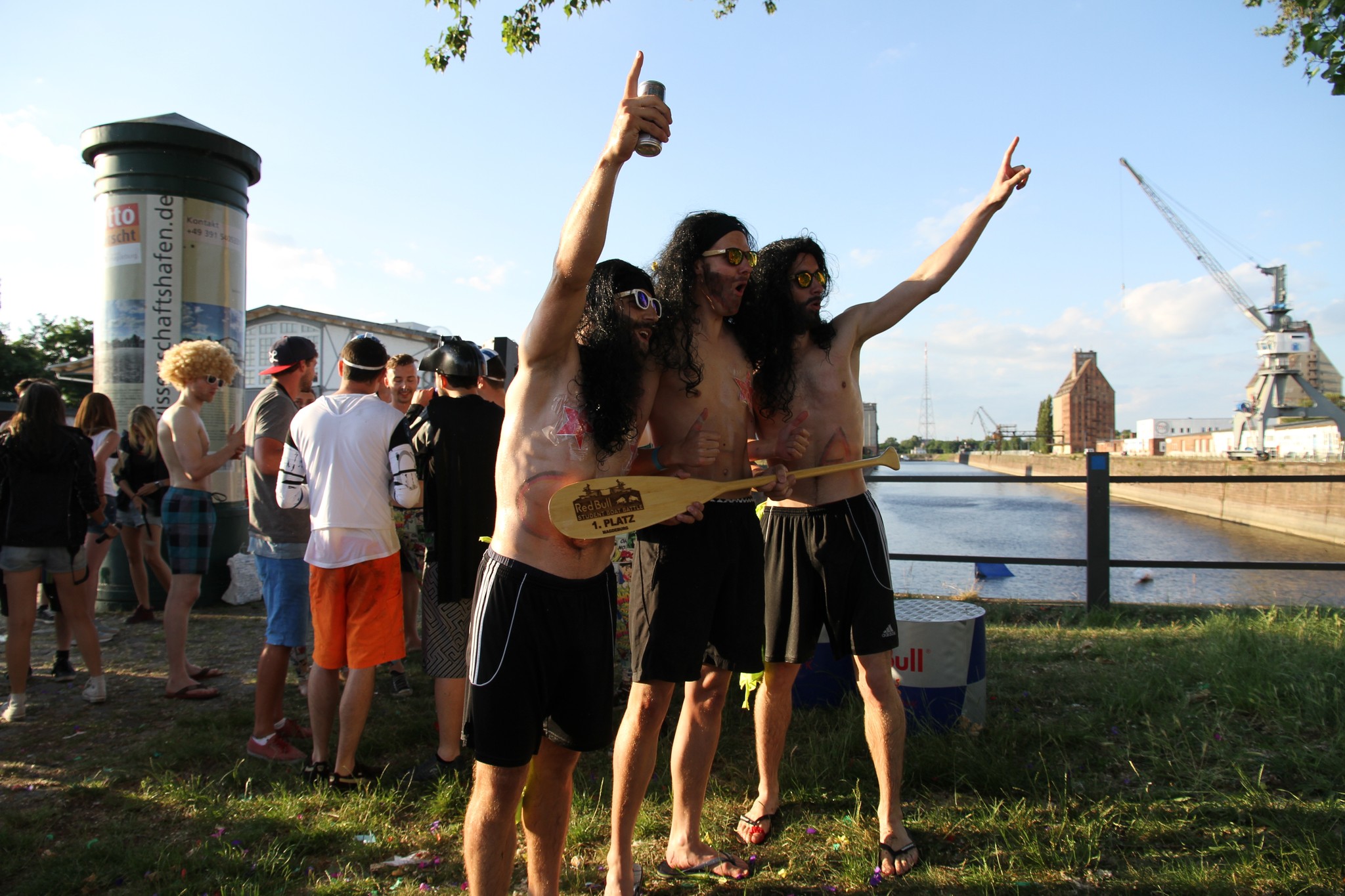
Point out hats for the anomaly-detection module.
[259,336,318,374]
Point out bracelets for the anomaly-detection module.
[651,445,668,471]
[130,494,136,499]
[155,481,162,489]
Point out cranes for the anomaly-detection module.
[1118,157,1345,461]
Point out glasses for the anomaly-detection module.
[701,247,758,270]
[788,267,827,290]
[615,288,663,318]
[205,374,224,388]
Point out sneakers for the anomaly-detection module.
[388,669,414,697]
[35,603,57,624]
[0,692,28,723]
[82,678,106,702]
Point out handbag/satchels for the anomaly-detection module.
[221,543,263,605]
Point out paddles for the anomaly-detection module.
[548,446,900,540]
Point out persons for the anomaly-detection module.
[733,135,1031,876]
[0,331,518,792]
[460,49,704,896]
[604,212,811,896]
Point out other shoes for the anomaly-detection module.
[275,718,313,738]
[69,618,120,646]
[301,754,330,783]
[4,664,32,681]
[396,756,464,783]
[246,731,308,765]
[124,605,155,627]
[49,658,78,682]
[329,759,381,791]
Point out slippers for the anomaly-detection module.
[631,861,644,896]
[736,812,775,846]
[189,665,224,681]
[878,840,920,878]
[656,850,751,882]
[162,680,220,700]
